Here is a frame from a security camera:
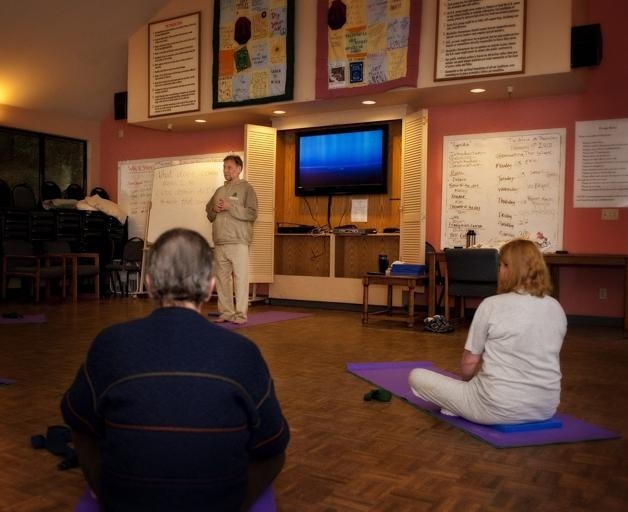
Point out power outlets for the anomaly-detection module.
[599,287,608,299]
[602,209,619,220]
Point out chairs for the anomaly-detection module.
[444,248,499,322]
[106,236,144,298]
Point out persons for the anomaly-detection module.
[60,227,289,512]
[409,240,568,425]
[206,155,257,325]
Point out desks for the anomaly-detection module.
[362,274,438,328]
[1,254,41,303]
[44,252,99,303]
[426,252,628,319]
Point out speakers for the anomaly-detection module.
[569,22,602,69]
[113,91,127,120]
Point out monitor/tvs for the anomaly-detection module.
[295,123,388,197]
